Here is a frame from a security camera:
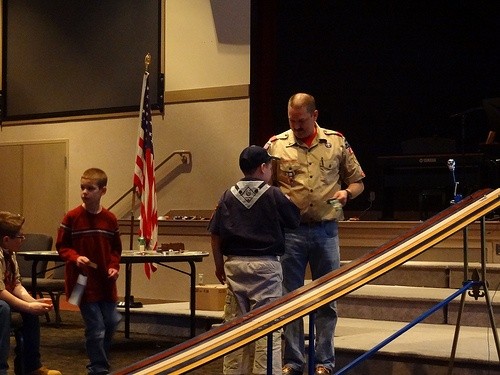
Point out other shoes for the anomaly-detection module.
[313,365,332,375]
[282,366,303,375]
[28,366,62,375]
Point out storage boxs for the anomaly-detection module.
[195,284,227,311]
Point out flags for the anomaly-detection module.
[133,73,157,279]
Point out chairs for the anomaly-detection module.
[18,262,65,327]
[17,234,53,322]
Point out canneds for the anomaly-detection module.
[137,237,147,251]
[198,274,204,285]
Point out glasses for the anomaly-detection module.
[15,234,24,239]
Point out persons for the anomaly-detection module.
[263,93,365,375]
[206,145,300,375]
[0,211,62,375]
[55,169,123,375]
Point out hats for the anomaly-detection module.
[239,146,279,173]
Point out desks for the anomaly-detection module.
[18,250,208,337]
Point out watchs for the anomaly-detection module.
[346,189,352,200]
